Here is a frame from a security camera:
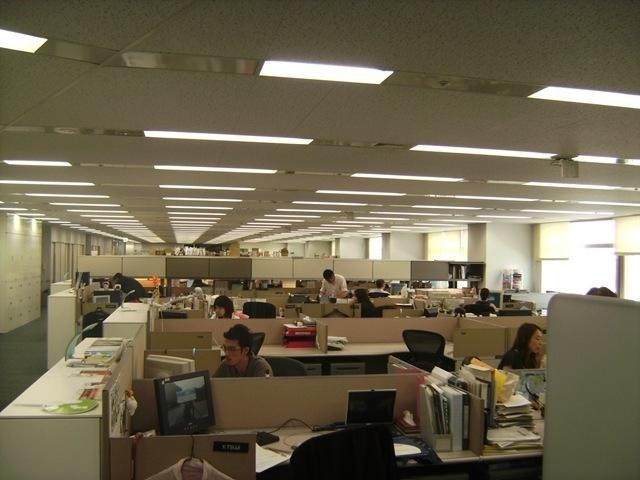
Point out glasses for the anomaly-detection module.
[220,346,244,353]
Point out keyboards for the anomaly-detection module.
[256,431,280,446]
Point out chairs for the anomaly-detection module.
[291,424,393,480]
[402,329,446,370]
[368,292,389,298]
[288,295,309,303]
[362,308,382,317]
[460,355,481,369]
[243,302,276,318]
[82,311,109,338]
[465,304,496,316]
[260,355,306,377]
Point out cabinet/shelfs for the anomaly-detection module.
[166,258,209,278]
[333,258,372,281]
[449,261,486,288]
[0,337,133,480]
[251,258,293,281]
[293,258,333,280]
[372,260,411,281]
[47,289,77,371]
[51,278,71,293]
[77,255,122,278]
[102,302,149,350]
[411,261,448,281]
[209,258,251,281]
[122,256,166,277]
[488,290,512,309]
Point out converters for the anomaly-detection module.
[332,421,345,429]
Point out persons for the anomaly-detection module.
[211,324,273,377]
[112,272,148,298]
[210,295,240,320]
[585,287,617,297]
[350,289,376,315]
[497,323,542,369]
[473,287,496,310]
[319,268,347,297]
[368,279,391,294]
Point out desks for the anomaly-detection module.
[511,292,557,310]
[101,347,480,479]
[452,369,546,480]
[318,317,507,361]
[146,318,327,359]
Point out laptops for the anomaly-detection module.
[345,389,403,437]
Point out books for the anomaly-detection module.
[84,337,123,362]
[418,357,544,456]
[410,290,432,310]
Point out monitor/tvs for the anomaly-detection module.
[478,289,504,311]
[154,369,216,435]
[75,272,90,286]
[158,310,188,318]
[424,307,439,318]
[92,290,123,303]
[229,282,244,290]
[390,283,408,296]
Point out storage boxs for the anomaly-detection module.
[304,363,323,375]
[328,363,366,375]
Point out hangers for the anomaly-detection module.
[184,435,204,464]
[322,308,348,317]
